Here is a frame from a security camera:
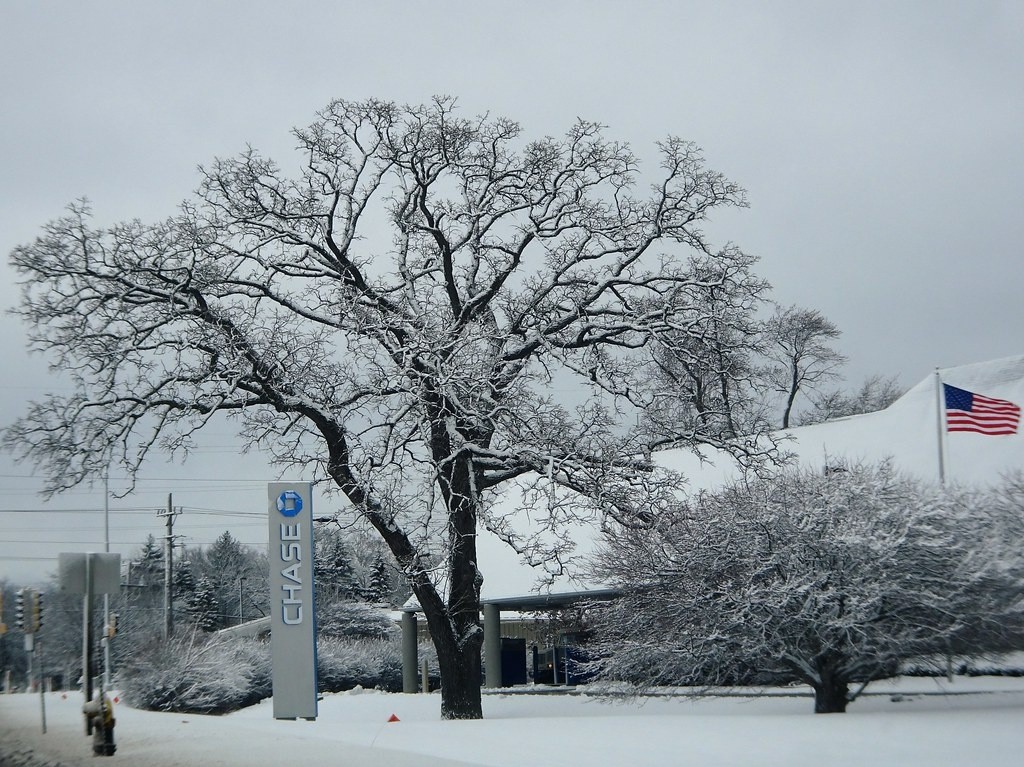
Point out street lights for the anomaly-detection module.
[239,576,247,624]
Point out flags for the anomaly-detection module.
[941,380,1020,436]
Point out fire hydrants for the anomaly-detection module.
[82,690,118,757]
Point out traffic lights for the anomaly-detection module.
[32,591,45,632]
[110,613,121,638]
[13,589,29,633]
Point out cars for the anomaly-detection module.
[529,648,554,684]
